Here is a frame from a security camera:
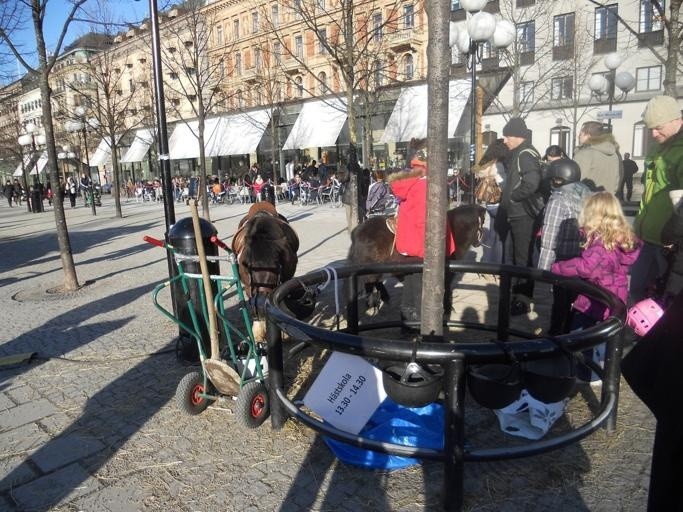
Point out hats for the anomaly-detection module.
[15,179,18,181]
[411,147,427,167]
[7,180,10,182]
[280,288,316,321]
[641,95,681,129]
[503,117,527,138]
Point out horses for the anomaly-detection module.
[346,203,487,326]
[232,200,301,344]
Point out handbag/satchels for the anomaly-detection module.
[474,160,502,204]
[512,161,552,218]
[493,390,569,440]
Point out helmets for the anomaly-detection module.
[376,361,446,408]
[468,359,524,409]
[523,354,576,404]
[550,159,581,188]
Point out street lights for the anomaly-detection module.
[448,1,517,203]
[18,124,47,212]
[588,53,637,133]
[64,106,100,216]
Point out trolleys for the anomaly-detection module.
[142,235,269,429]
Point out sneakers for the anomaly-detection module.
[564,375,604,387]
[511,299,530,316]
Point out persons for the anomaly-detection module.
[126,157,404,218]
[1,172,92,208]
[387,94,683,388]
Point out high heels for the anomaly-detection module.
[494,274,501,282]
[478,272,495,282]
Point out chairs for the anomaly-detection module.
[133,175,344,211]
